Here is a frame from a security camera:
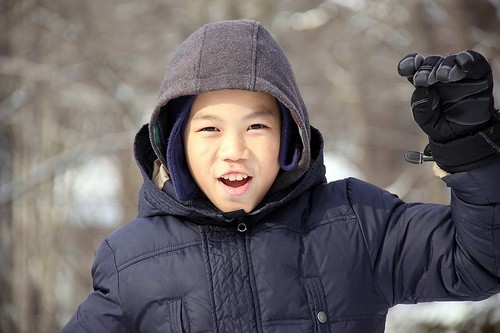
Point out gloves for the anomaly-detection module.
[398,49,500,173]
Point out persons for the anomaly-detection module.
[62,18,500,333]
